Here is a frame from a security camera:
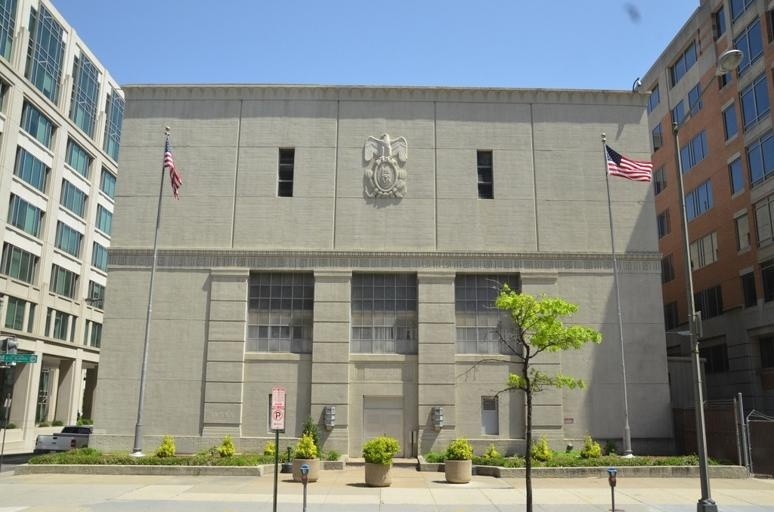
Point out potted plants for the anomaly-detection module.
[293,430,320,482]
[362,437,401,486]
[445,439,473,483]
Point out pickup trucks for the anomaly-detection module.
[33,426,92,454]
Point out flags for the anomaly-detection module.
[163,138,183,201]
[606,145,654,184]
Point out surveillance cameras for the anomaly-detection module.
[637,81,642,87]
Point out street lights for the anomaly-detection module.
[671,50,743,512]
